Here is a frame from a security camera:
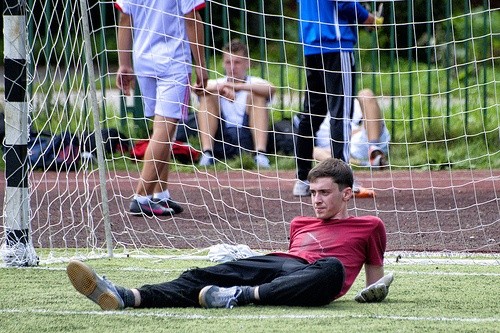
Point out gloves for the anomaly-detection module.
[355,272,394,302]
[372,16,384,30]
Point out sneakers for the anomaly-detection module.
[254,154,271,170]
[67,260,125,311]
[130,198,175,216]
[199,152,215,167]
[153,199,184,213]
[198,285,241,308]
[293,181,310,196]
[352,185,377,198]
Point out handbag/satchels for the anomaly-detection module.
[132,139,198,165]
[27,131,79,172]
[82,129,129,157]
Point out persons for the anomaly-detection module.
[312,89,390,170]
[194,39,276,167]
[111,0,209,216]
[291,0,383,198]
[67,157,396,311]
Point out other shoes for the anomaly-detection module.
[369,147,387,170]
